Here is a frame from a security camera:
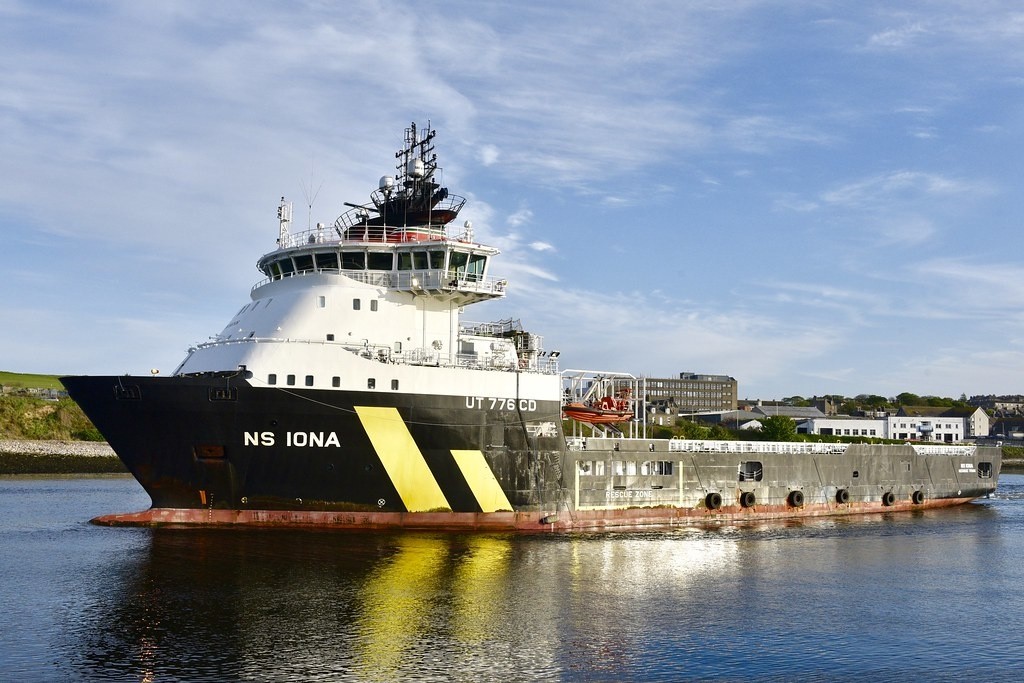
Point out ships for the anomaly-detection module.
[56,119,1003,539]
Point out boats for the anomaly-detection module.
[557,389,638,424]
